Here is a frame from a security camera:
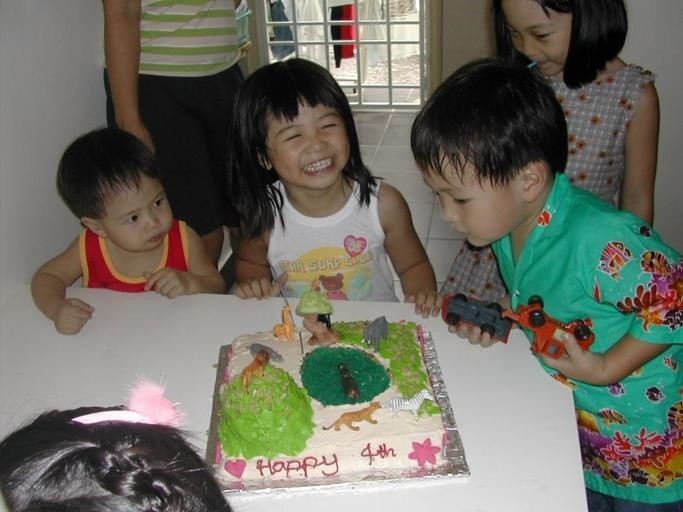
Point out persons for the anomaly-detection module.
[227,58,444,318]
[30,127,227,335]
[438,0,659,302]
[411,59,682,511]
[0,402,236,512]
[97,0,248,293]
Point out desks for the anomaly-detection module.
[0,281,591,512]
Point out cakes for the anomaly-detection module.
[211,291,452,491]
